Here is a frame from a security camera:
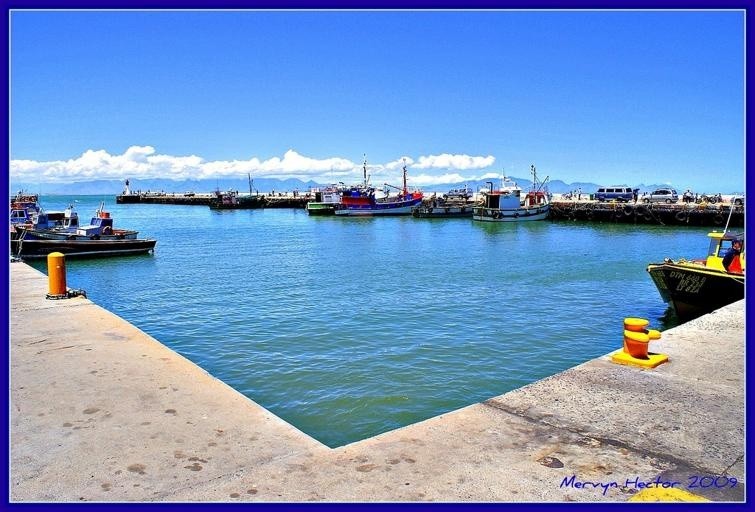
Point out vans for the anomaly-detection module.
[595,187,633,203]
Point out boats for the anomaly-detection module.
[646,231,746,325]
[210,157,551,222]
[10,189,157,263]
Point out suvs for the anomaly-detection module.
[641,186,679,204]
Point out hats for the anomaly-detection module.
[732,239,742,245]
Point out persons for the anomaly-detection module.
[559,181,726,206]
[722,239,743,273]
